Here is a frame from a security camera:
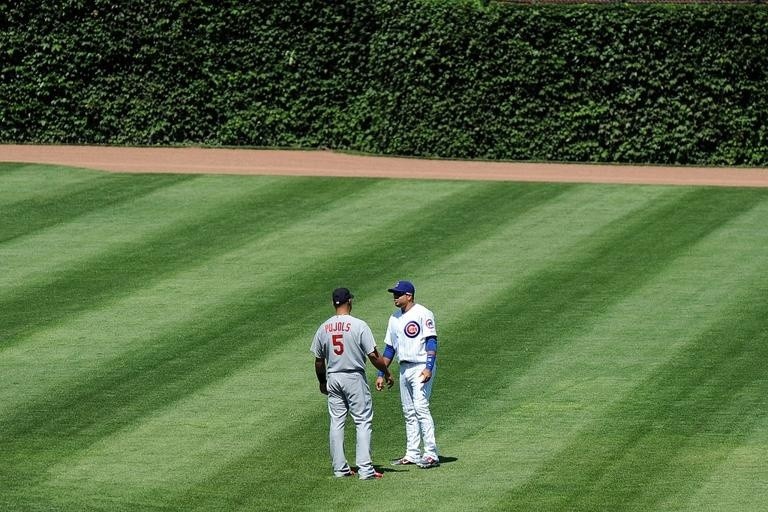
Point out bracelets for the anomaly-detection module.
[377,370,383,378]
[426,353,436,372]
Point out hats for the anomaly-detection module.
[388,280,415,294]
[333,288,354,306]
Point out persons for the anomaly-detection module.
[375,279,440,468]
[310,288,394,481]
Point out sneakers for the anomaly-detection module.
[359,472,383,480]
[416,455,439,467]
[335,470,355,477]
[394,456,413,464]
